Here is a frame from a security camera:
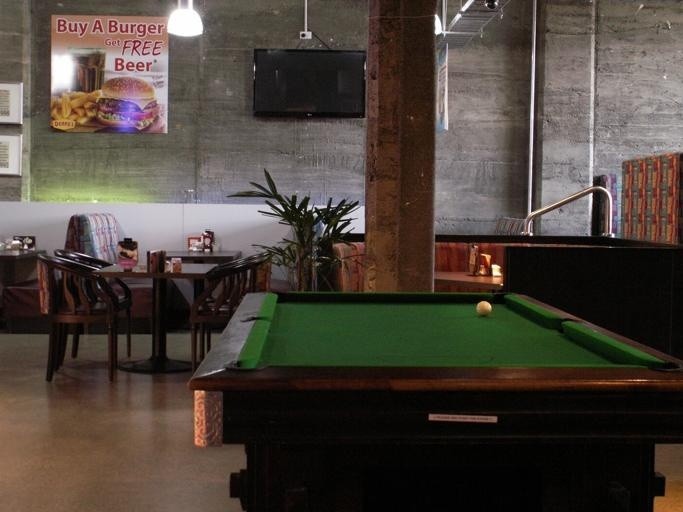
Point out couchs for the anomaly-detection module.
[2,212,154,330]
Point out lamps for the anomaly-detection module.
[166,0,204,36]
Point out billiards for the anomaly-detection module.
[477,300,493,316]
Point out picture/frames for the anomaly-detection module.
[0,79,23,178]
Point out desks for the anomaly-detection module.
[186,290,683,512]
[434,272,504,293]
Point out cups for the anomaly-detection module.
[67,47,107,93]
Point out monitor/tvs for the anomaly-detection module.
[253,48,367,118]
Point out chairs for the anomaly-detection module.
[37,248,274,383]
